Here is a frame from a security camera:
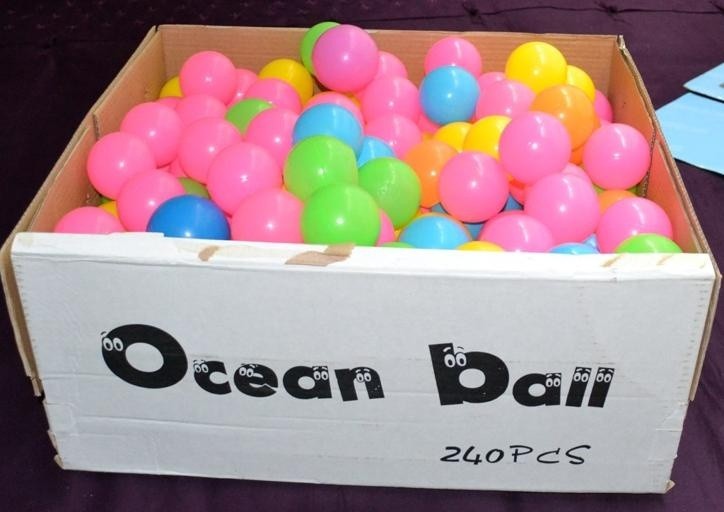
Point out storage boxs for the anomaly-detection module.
[0,22,722,495]
